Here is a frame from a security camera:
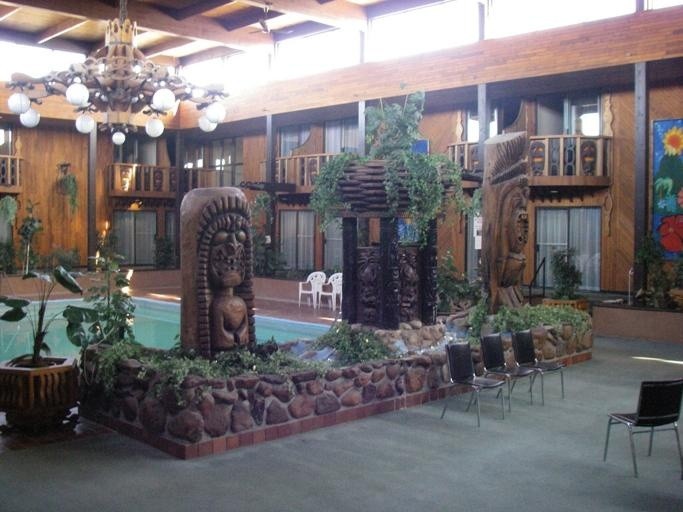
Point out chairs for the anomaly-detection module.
[298,271,344,315]
[601,376,683,481]
[438,328,567,427]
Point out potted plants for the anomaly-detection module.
[0,241,93,435]
[539,245,589,316]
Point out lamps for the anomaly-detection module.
[4,1,233,146]
[128,197,144,211]
